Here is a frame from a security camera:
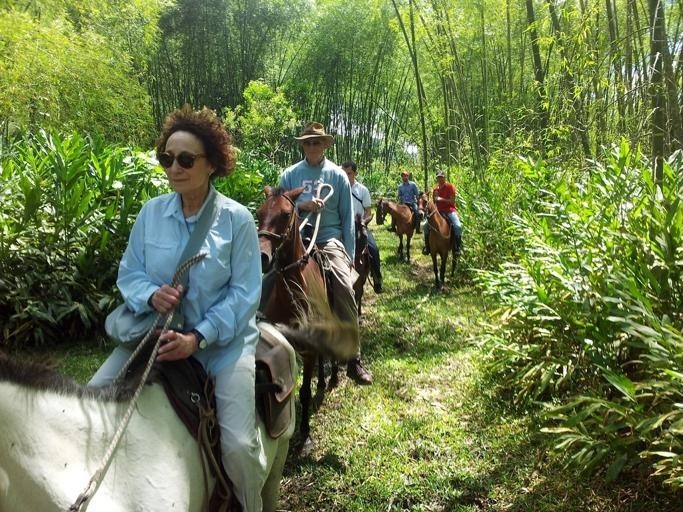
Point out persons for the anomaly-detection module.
[422,170,462,257]
[342,161,385,294]
[275,121,373,385]
[387,171,423,234]
[85,102,268,512]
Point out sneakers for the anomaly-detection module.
[388,228,396,233]
[415,230,423,235]
[373,283,384,294]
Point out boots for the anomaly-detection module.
[455,234,461,257]
[423,233,430,255]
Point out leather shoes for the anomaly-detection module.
[347,360,373,386]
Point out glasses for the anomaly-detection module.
[302,140,324,148]
[159,151,209,169]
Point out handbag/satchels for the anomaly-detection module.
[103,304,186,353]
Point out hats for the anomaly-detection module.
[293,121,335,148]
[401,171,408,176]
[436,171,446,177]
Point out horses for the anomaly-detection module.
[374,198,416,265]
[415,191,459,290]
[0,317,361,512]
[353,213,375,326]
[256,184,339,458]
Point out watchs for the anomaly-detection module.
[191,328,207,353]
